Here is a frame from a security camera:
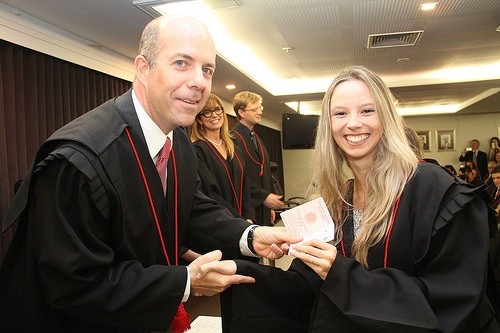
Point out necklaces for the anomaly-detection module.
[210,139,223,145]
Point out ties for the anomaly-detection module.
[156,137,171,197]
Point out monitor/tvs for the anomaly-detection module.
[282,113,320,149]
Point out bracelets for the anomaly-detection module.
[247,226,259,254]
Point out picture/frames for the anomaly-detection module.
[436,129,457,151]
[416,131,432,151]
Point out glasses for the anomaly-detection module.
[243,106,263,112]
[198,106,223,118]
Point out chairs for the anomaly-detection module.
[271,161,307,213]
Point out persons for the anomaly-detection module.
[229,90,286,226]
[487,137,500,229]
[459,139,488,184]
[0,14,305,333]
[193,94,259,224]
[442,136,451,147]
[195,65,490,333]
